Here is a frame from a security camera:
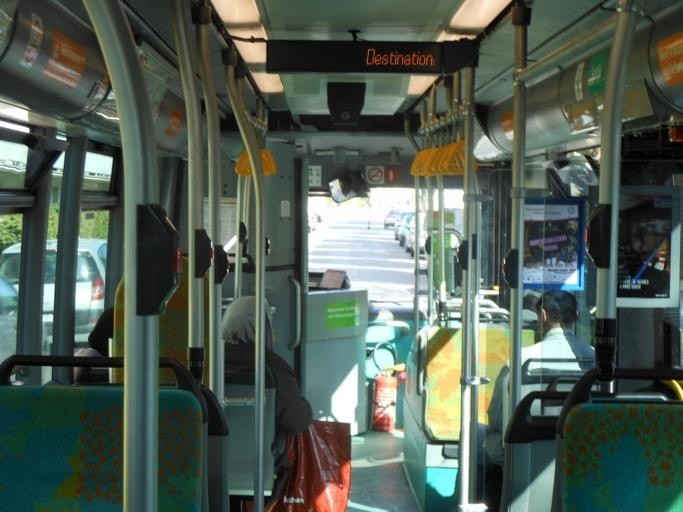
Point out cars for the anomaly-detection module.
[383,206,430,274]
[0,276,51,380]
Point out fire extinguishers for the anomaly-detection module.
[370,341,398,432]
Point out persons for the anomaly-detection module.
[334,175,359,201]
[222,295,313,500]
[478,291,601,508]
[71,306,112,387]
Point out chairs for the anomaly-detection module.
[1,313,300,511]
[393,312,683,512]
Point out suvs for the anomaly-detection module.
[0,237,108,349]
